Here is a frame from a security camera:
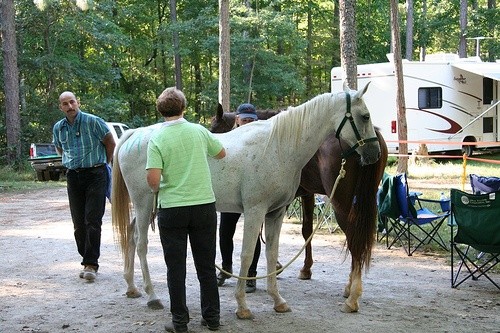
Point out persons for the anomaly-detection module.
[145,86,226,333]
[217,103,261,293]
[53,91,116,280]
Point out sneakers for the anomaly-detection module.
[79,266,97,280]
[165,323,189,333]
[244,279,256,294]
[200,318,221,331]
[217,270,233,286]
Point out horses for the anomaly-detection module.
[110,80,382,320]
[210,103,388,314]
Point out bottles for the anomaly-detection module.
[439,192,449,200]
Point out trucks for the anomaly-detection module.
[330,53,500,158]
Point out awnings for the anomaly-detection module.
[451,61,500,138]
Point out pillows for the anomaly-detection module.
[469,174,500,195]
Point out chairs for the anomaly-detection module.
[288,170,450,256]
[451,188,500,291]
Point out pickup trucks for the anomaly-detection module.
[26,122,130,181]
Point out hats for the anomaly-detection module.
[235,103,258,120]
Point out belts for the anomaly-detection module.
[76,165,106,172]
